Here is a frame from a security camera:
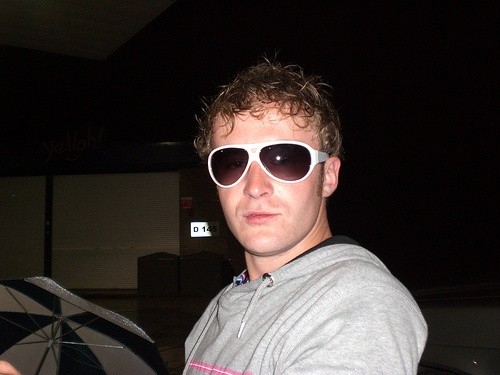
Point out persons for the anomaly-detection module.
[0,60,428,375]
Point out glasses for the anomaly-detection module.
[207,141,329,187]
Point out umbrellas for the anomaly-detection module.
[0,277,168,375]
